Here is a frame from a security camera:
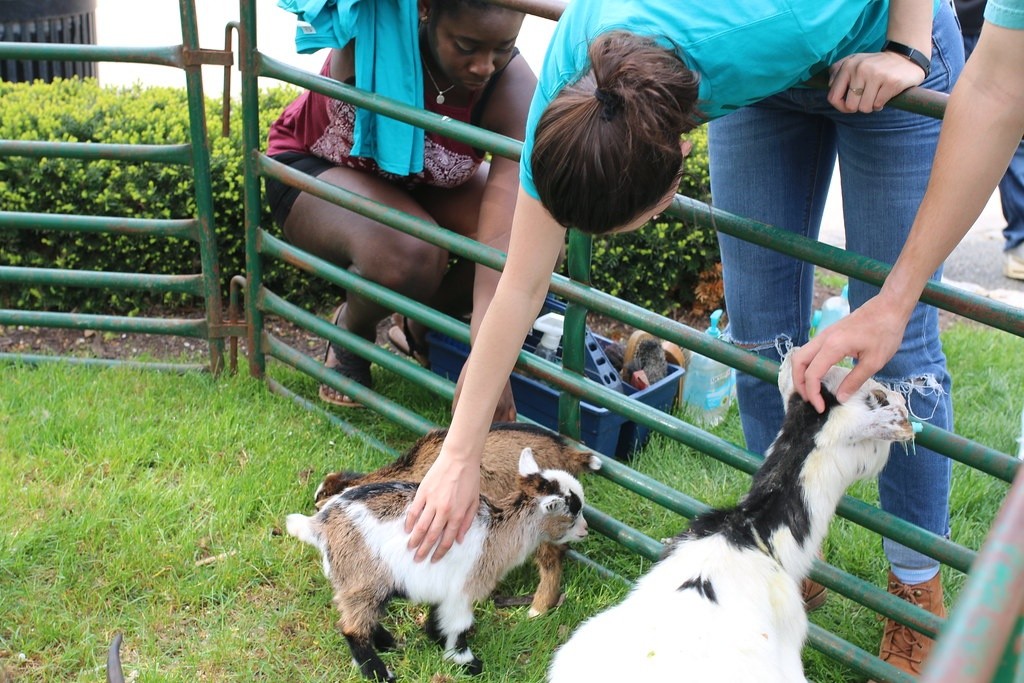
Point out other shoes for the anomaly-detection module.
[864,568,948,683]
[1002,239,1024,280]
[801,547,828,613]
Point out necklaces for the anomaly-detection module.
[421,53,455,104]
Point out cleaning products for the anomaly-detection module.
[531,311,564,363]
[681,284,850,430]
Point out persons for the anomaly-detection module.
[265,0,538,424]
[403,0,966,682]
[954,0,1024,281]
[792,0,1024,415]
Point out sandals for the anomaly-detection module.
[387,311,431,372]
[318,301,373,408]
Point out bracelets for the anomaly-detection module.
[881,39,932,78]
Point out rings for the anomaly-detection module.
[849,87,863,94]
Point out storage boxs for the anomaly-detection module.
[426,298,686,465]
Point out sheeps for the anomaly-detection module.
[547,347,916,683]
[285,425,602,683]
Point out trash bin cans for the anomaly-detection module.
[0,0,100,89]
